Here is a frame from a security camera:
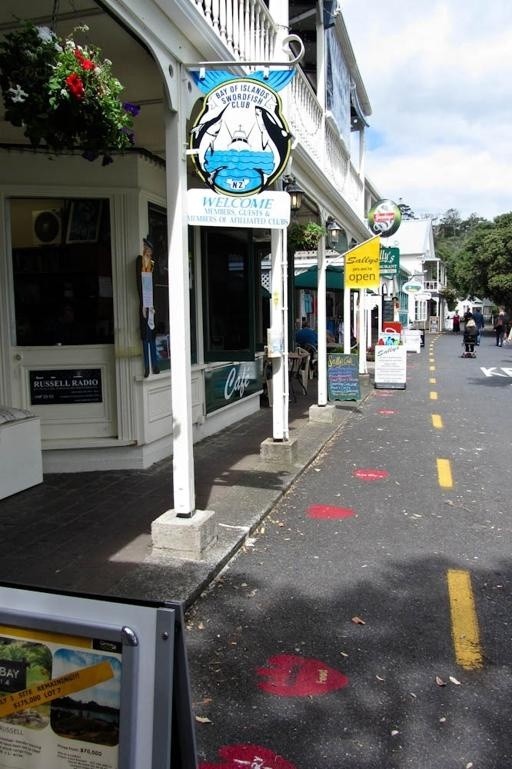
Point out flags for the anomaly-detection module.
[345,237,380,288]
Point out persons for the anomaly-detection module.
[294,322,317,370]
[453,305,512,358]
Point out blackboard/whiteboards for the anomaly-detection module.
[326,353,359,400]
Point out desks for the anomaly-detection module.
[288,352,311,402]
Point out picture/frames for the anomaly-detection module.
[64,199,104,244]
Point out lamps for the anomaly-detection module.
[282,174,304,212]
[327,216,343,246]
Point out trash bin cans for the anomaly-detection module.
[295,330,317,360]
[327,321,335,335]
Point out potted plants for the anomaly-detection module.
[288,220,324,250]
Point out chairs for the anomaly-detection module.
[295,342,318,395]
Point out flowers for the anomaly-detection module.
[1,24,139,165]
